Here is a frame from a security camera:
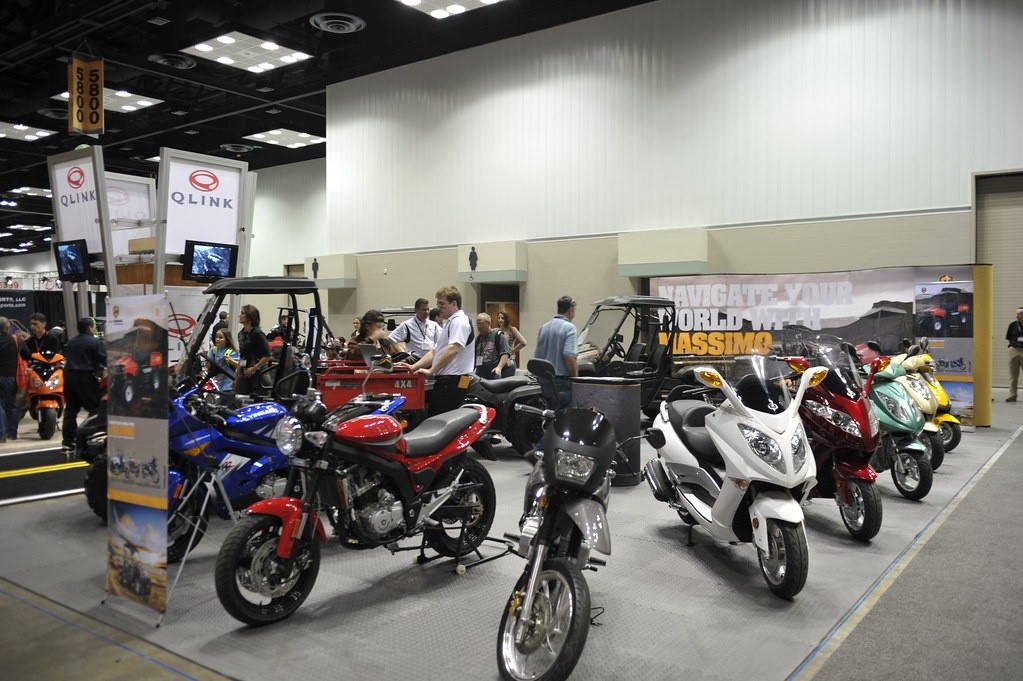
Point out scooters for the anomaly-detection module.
[22,348,68,442]
[638,345,833,600]
[757,336,963,546]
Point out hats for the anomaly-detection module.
[360,309,384,324]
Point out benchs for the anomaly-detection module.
[234,353,313,409]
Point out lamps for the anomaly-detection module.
[5,276,12,285]
[41,275,50,284]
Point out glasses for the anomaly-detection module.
[567,298,576,308]
[435,302,450,309]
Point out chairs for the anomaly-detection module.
[623,344,645,372]
[626,344,668,380]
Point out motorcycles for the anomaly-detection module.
[496,357,667,681]
[211,340,497,628]
[67,274,691,563]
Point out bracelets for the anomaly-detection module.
[249,367,255,374]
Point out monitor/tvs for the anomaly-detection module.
[182,240,238,283]
[52,239,92,282]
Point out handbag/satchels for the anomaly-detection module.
[15,353,27,392]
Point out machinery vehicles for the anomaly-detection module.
[919,292,972,339]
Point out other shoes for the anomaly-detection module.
[1005,396,1017,402]
[7,433,18,440]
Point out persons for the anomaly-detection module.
[206,328,240,391]
[345,308,527,380]
[212,310,228,345]
[524,296,578,467]
[0,313,66,443]
[231,305,271,396]
[62,317,107,447]
[393,286,475,417]
[1005,306,1023,402]
[266,315,346,347]
